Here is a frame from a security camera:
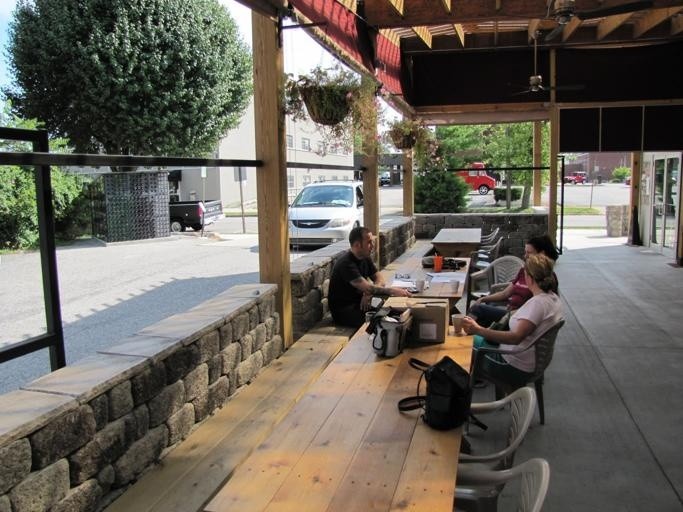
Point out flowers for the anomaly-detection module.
[275,59,387,158]
[385,114,433,160]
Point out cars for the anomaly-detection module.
[287,179,364,251]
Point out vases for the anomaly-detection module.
[298,85,352,126]
[389,130,416,149]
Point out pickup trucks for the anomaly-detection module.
[169,199,224,232]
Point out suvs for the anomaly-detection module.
[564,171,587,185]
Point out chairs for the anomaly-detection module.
[470,227,505,270]
[453,385,552,511]
[466,255,566,425]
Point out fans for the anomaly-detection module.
[504,30,585,96]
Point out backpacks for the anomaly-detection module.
[398,356,472,429]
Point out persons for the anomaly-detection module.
[468,236,558,328]
[462,253,563,401]
[328,227,413,330]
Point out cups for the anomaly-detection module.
[451,313,465,332]
[415,278,425,293]
[448,279,459,294]
[364,310,377,341]
[432,256,443,272]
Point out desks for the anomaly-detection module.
[387,227,483,308]
[206,320,475,512]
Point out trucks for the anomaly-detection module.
[456,162,498,196]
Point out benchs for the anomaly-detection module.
[99,236,435,512]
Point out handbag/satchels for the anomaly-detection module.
[365,306,415,358]
[484,311,511,346]
[422,257,466,269]
